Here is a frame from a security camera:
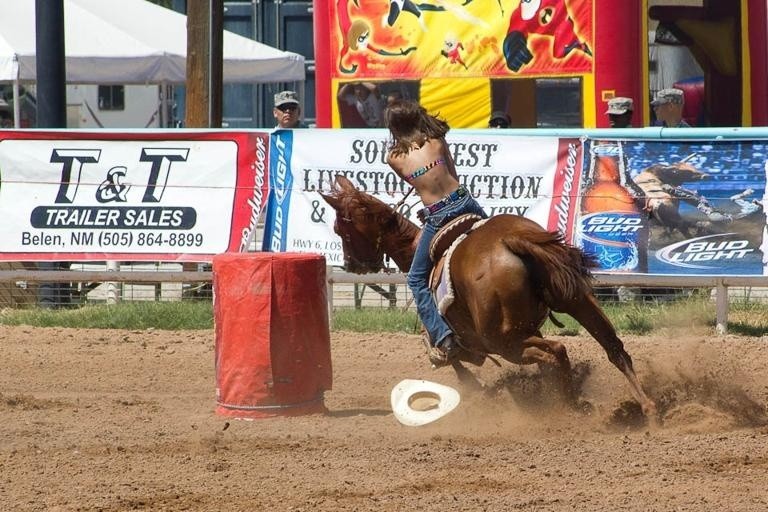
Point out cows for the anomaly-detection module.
[632,152,710,239]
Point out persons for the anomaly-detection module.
[663,183,762,232]
[272,90,309,128]
[649,88,691,127]
[488,111,512,129]
[605,98,635,128]
[382,99,489,371]
[337,83,384,128]
[382,90,403,128]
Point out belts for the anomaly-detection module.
[416,184,467,223]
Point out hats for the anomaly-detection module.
[490,110,512,125]
[389,378,462,429]
[649,88,684,108]
[273,90,300,108]
[604,96,635,117]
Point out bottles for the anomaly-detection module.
[579,140,651,273]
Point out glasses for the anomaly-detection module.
[489,119,509,128]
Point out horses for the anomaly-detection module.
[316,174,664,432]
